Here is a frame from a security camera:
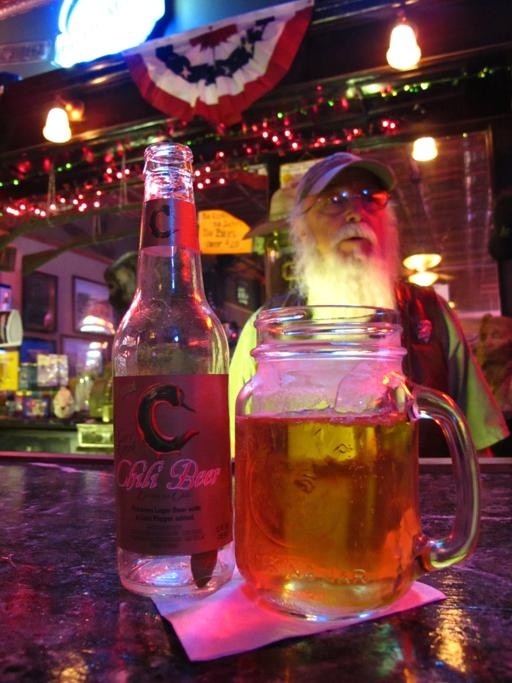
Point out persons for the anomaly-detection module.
[227,151,512,460]
[475,312,512,457]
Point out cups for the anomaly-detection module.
[233,304,483,620]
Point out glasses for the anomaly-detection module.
[314,185,390,216]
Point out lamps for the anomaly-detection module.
[385,0,421,72]
[42,94,71,143]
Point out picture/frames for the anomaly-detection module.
[21,271,117,375]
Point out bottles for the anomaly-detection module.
[109,141,234,601]
[1,351,116,425]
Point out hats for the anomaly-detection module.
[296,153,397,214]
